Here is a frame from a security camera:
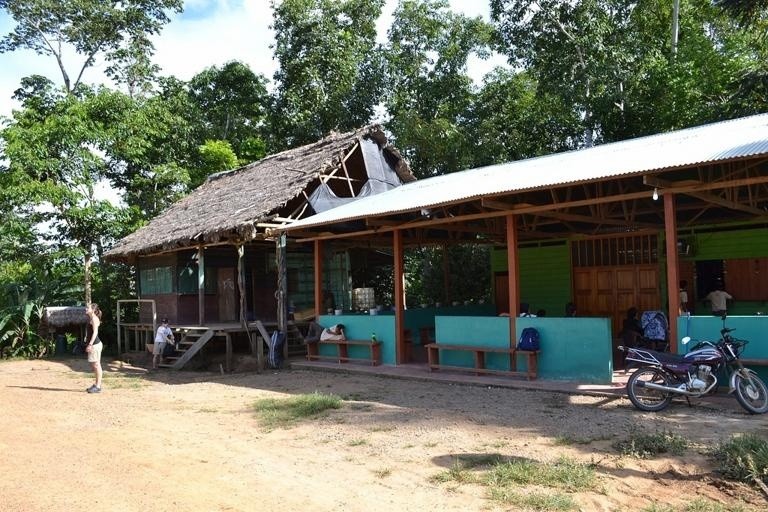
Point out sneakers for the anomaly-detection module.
[85,385,101,394]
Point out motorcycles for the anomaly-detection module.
[617,311,767,414]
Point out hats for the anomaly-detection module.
[161,318,169,324]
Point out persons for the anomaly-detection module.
[678,279,689,317]
[620,307,657,366]
[83,303,104,395]
[703,281,734,316]
[153,318,175,371]
[563,302,577,318]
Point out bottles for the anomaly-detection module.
[372,332,376,344]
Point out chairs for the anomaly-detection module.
[617,327,645,366]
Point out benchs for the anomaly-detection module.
[730,359,768,367]
[303,340,381,366]
[424,343,541,381]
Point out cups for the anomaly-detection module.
[369,309,377,316]
[335,309,343,315]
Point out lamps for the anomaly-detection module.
[652,187,659,201]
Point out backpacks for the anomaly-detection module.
[516,327,541,353]
